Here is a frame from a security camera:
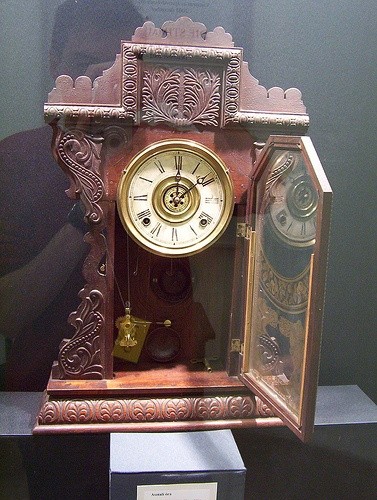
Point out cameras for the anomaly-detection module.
[59,115,136,155]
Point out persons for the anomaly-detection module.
[1,1,204,500]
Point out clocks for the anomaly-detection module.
[116,137,237,259]
[266,150,318,248]
